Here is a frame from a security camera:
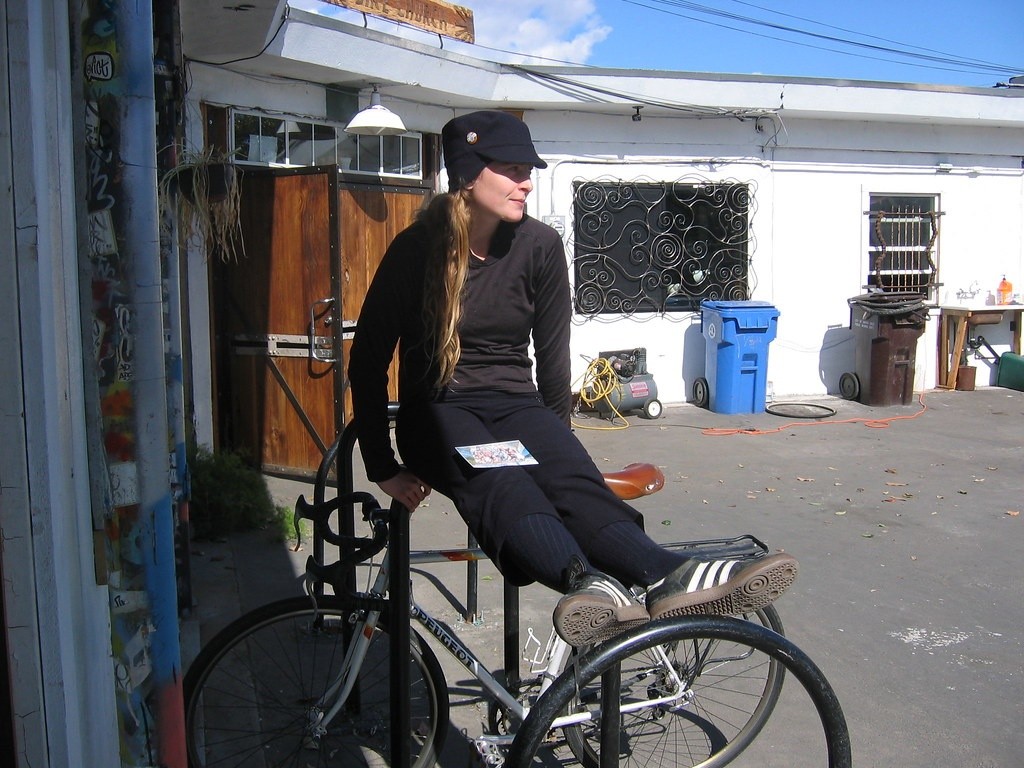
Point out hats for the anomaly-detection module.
[442,111,549,184]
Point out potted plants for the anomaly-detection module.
[158,136,246,264]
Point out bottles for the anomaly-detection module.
[997,274,1012,304]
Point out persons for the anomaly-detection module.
[347,110,799,646]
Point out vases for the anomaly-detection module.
[957,365,977,391]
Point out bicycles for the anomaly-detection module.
[181,461,788,768]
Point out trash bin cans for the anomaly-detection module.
[693,300,781,415]
[996,351,1024,391]
[838,292,930,407]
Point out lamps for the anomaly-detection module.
[343,84,407,136]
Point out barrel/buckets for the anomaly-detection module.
[956,365,977,391]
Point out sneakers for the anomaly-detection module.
[552,554,650,646]
[646,553,800,623]
